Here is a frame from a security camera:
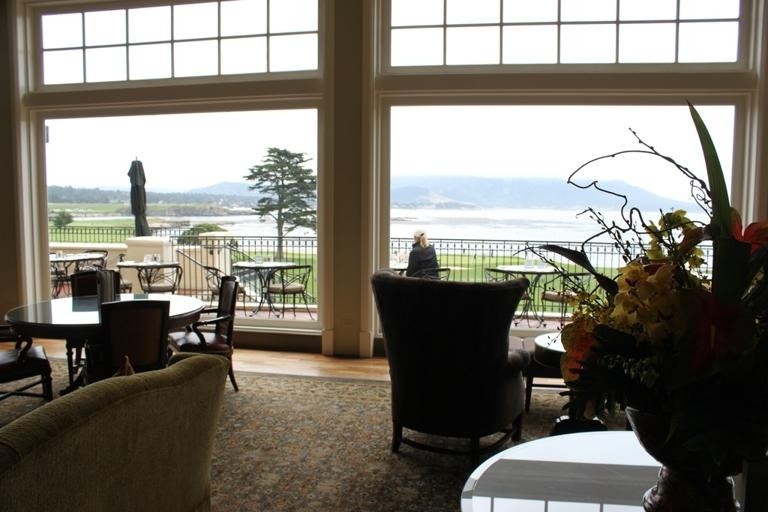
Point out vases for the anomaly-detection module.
[625,406,768,511]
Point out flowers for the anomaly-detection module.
[488,91,768,425]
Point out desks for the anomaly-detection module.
[460,431,741,512]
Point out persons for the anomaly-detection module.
[406,230,440,280]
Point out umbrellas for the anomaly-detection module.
[128,156,152,236]
